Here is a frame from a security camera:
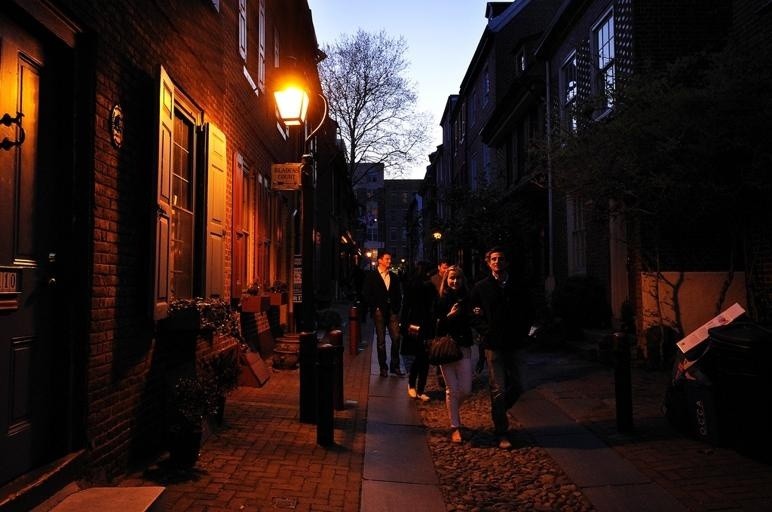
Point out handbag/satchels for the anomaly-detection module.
[428,335,464,365]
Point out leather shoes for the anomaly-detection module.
[407,383,417,398]
[416,393,431,402]
[451,429,462,443]
[379,367,389,378]
[390,366,405,376]
[498,439,513,449]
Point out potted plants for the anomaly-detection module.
[267,279,288,306]
[241,279,270,313]
[163,296,247,473]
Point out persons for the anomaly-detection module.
[429,264,483,445]
[365,251,406,378]
[397,259,441,403]
[429,260,452,392]
[469,247,536,450]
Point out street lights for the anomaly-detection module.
[431,228,446,265]
[269,50,333,425]
[365,249,372,270]
